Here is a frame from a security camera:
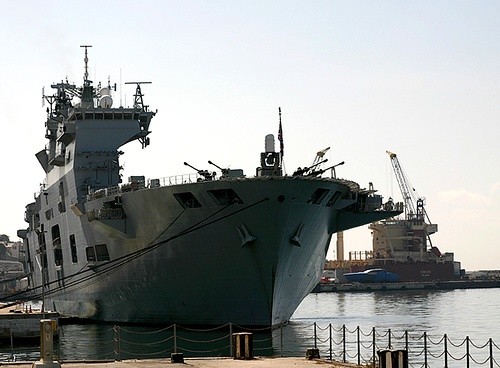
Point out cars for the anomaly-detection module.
[341,269,400,284]
[321,270,341,284]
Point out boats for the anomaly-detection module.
[323,150,500,280]
[16,44,406,338]
[0,301,61,339]
